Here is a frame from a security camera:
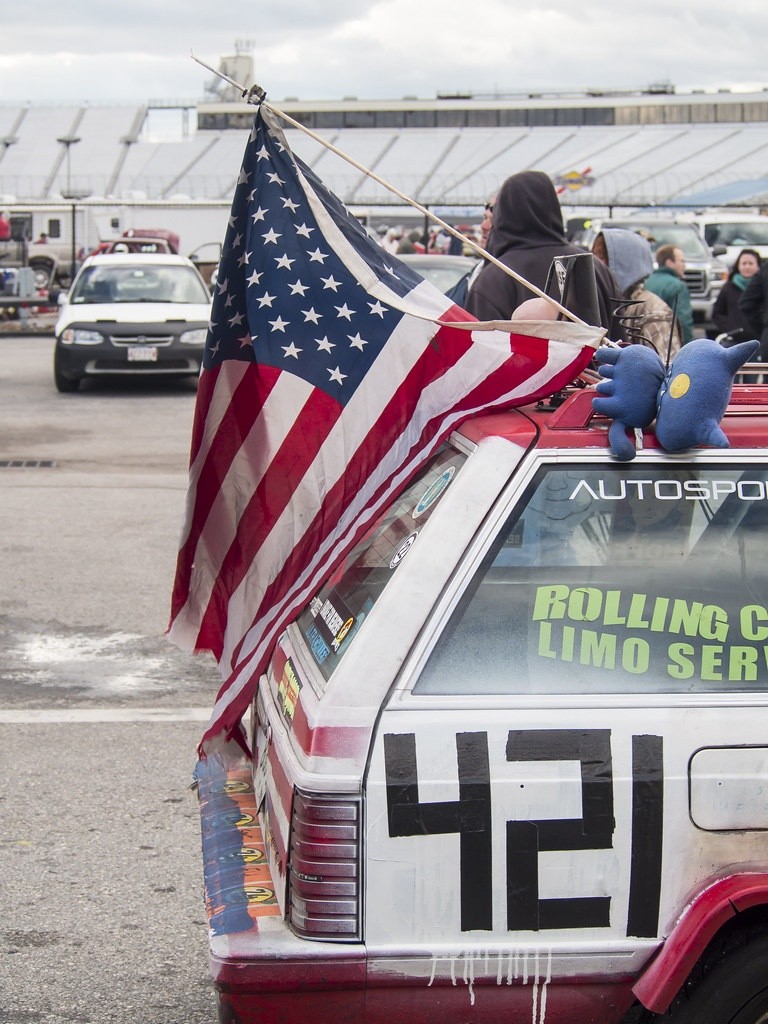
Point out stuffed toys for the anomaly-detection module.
[591,337,761,461]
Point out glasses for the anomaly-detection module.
[484,202,494,214]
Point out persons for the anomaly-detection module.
[463,171,628,342]
[376,224,480,257]
[738,261,768,384]
[467,195,497,292]
[590,228,680,362]
[645,244,696,345]
[712,249,763,383]
[38,233,47,244]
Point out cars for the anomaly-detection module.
[52,239,224,394]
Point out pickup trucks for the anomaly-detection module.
[0,240,86,291]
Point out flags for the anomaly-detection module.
[162,106,608,761]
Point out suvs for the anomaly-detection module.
[579,217,728,326]
[184,384,768,1022]
[686,214,768,270]
